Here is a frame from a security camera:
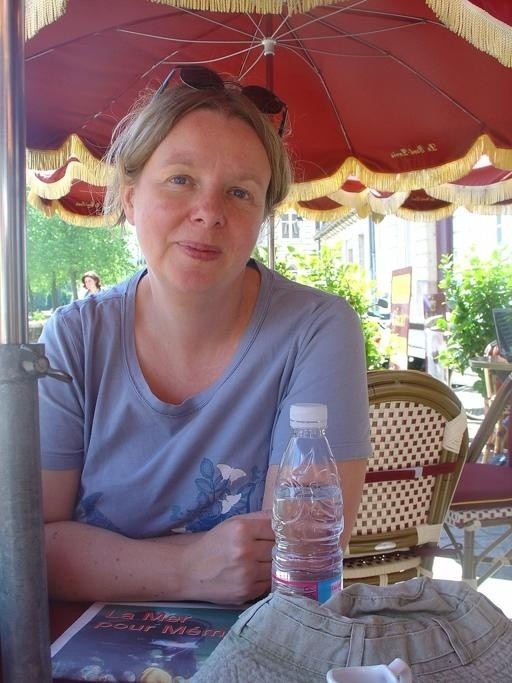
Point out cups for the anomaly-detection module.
[324,656,416,683]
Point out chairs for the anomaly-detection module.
[340,369,469,588]
[434,371,512,588]
[483,342,512,465]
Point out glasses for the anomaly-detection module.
[151,63,289,137]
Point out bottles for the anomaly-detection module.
[269,400,346,607]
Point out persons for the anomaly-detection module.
[36,63,373,607]
[82,271,101,297]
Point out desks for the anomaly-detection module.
[469,357,512,466]
